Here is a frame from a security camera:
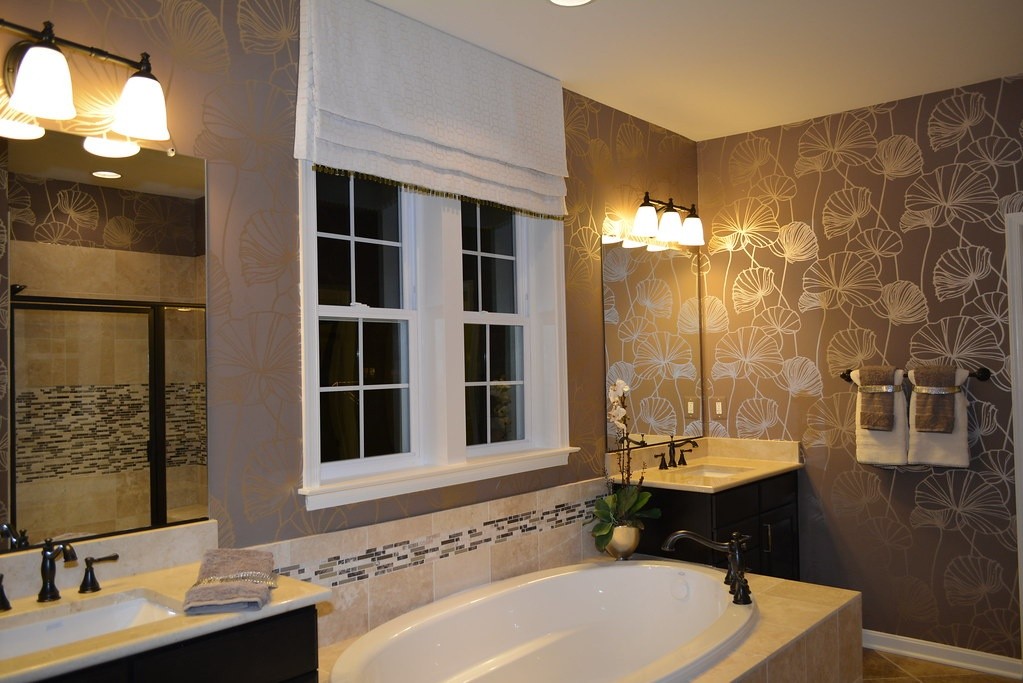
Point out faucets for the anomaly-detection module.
[618,433,647,447]
[667,433,699,468]
[36,537,78,603]
[0,523,30,551]
[662,529,750,596]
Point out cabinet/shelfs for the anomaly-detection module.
[33,603,318,683]
[612,471,800,580]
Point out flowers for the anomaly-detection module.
[587,377,650,552]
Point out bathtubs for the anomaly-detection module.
[318,549,864,683]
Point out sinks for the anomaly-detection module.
[666,465,758,478]
[0,588,184,657]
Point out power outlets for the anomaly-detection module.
[710,396,727,421]
[684,396,698,419]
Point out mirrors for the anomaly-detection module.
[600,236,703,454]
[0,118,209,556]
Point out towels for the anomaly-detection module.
[34,532,98,546]
[849,365,910,470]
[183,549,278,616]
[907,365,971,468]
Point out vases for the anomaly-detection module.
[605,523,642,559]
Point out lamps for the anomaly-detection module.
[629,192,706,246]
[0,18,171,141]
